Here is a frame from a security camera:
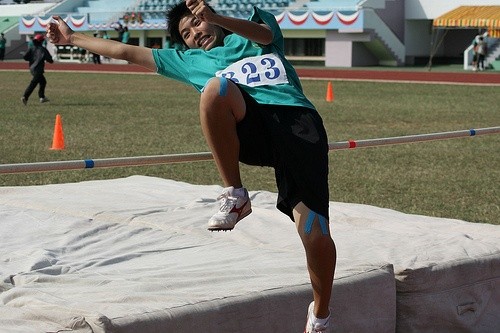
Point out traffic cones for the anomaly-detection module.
[326,81,334,100]
[48,114,66,150]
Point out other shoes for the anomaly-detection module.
[21,97,27,105]
[40,97,46,102]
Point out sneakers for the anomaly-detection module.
[208,186,252,232]
[303,301,331,333]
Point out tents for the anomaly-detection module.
[428,6,500,71]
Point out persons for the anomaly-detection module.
[46,0,336,333]
[21,33,54,106]
[472,32,486,72]
[78,24,130,64]
[0,33,6,61]
